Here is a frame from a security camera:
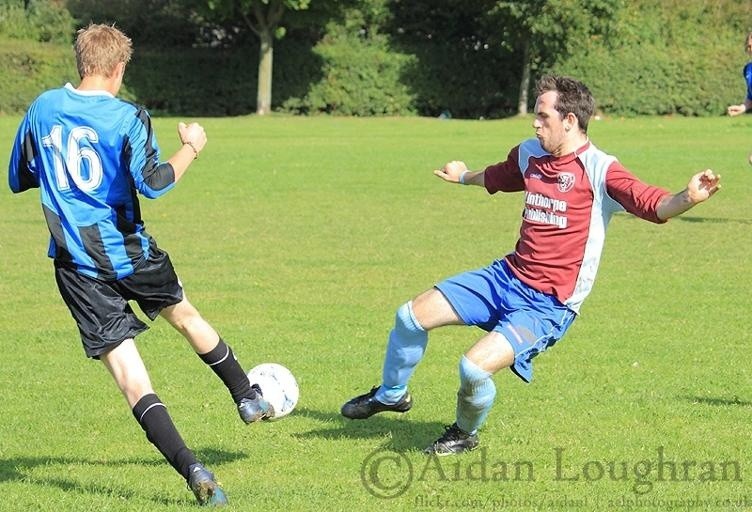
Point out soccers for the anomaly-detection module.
[249,362,301,419]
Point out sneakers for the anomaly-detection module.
[341,386,412,421]
[424,423,480,458]
[237,384,271,425]
[189,463,229,507]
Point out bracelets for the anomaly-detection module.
[458,169,472,188]
[179,141,200,164]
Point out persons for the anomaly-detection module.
[333,73,723,459]
[7,14,273,510]
[725,31,751,166]
